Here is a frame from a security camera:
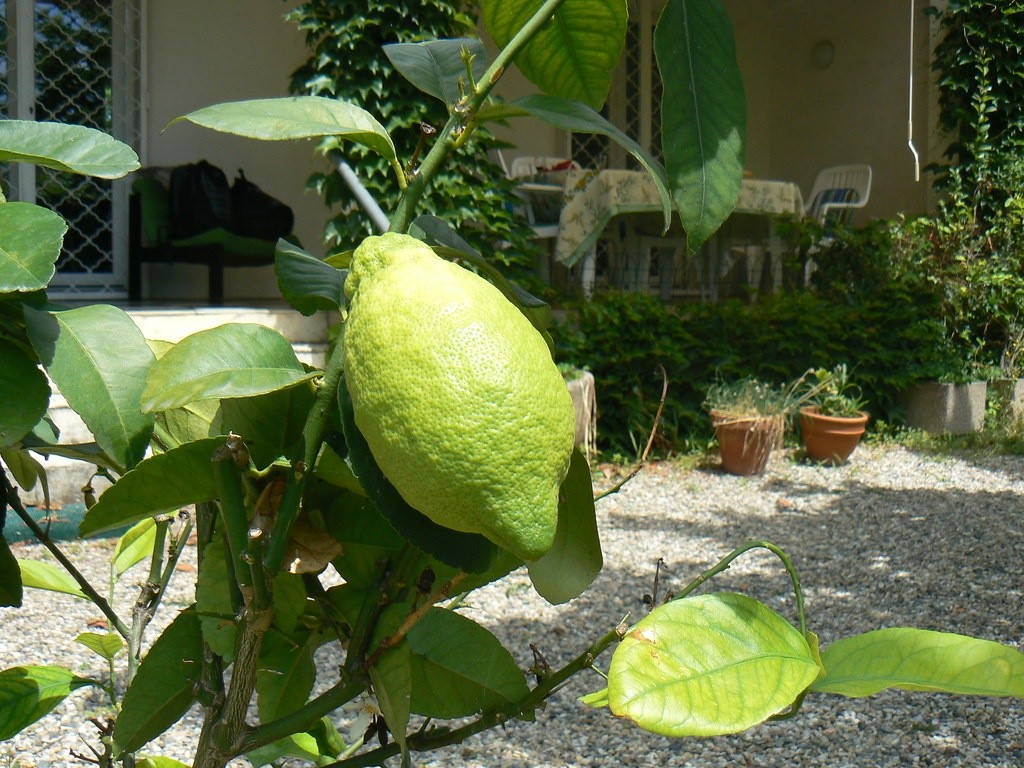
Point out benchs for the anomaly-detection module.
[128,177,304,305]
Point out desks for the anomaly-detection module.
[556,168,807,301]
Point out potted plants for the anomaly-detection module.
[702,373,793,477]
[798,364,870,466]
[883,205,1024,437]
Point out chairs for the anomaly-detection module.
[512,156,706,304]
[804,164,872,281]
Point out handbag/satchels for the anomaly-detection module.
[230,167,294,242]
[170,160,233,242]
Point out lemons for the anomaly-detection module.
[344,231,576,561]
